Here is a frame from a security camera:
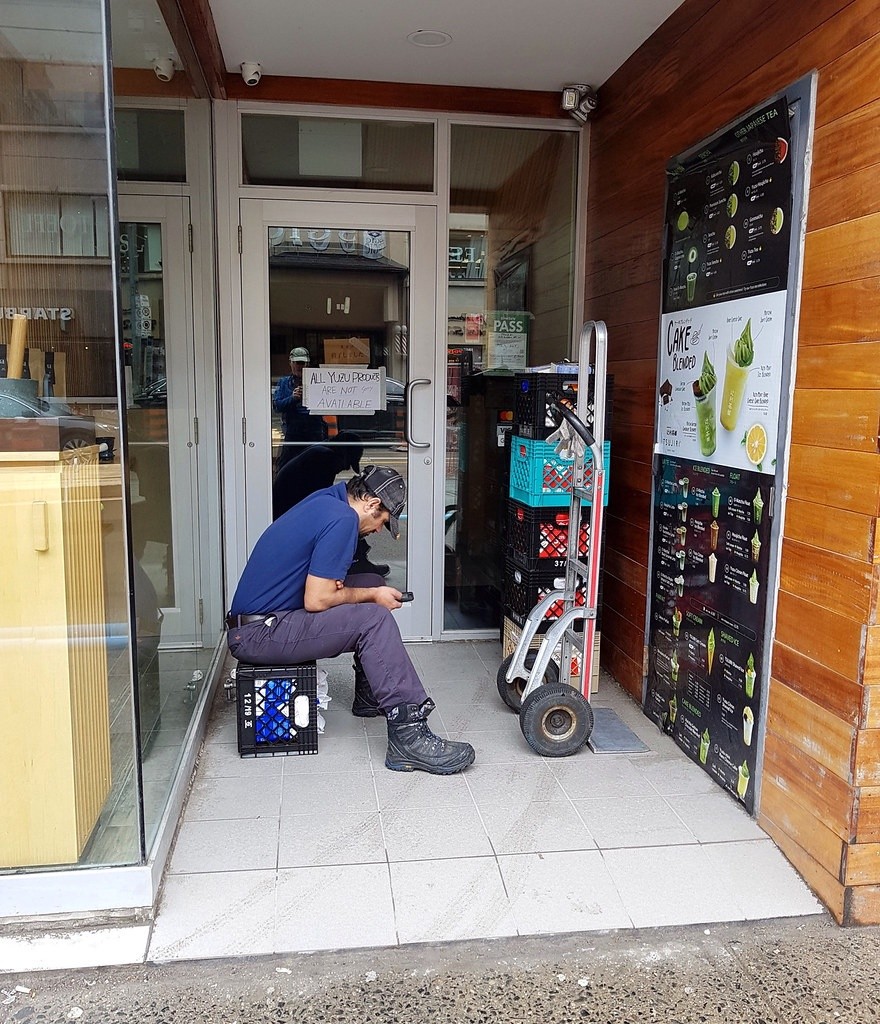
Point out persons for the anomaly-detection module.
[273,346,312,474]
[222,465,475,776]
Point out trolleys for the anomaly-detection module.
[493,320,651,758]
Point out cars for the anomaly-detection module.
[270,377,406,443]
[0,388,117,466]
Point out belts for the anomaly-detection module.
[226,614,267,630]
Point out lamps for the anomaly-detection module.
[561,84,597,126]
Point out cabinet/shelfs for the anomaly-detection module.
[0,412,113,868]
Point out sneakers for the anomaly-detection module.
[385,697,475,775]
[352,664,385,716]
[347,559,391,577]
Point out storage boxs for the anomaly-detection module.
[504,499,606,571]
[510,436,611,507]
[503,616,601,695]
[512,371,613,440]
[502,552,601,633]
[237,657,318,756]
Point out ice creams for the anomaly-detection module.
[734,317,755,367]
[699,350,718,395]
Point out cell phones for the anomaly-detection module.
[395,592,415,602]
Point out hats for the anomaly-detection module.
[332,429,363,475]
[361,464,406,542]
[289,347,310,362]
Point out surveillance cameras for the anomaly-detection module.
[152,58,174,81]
[560,88,598,126]
[241,62,261,85]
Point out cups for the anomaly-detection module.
[679,550,685,570]
[743,718,753,746]
[678,579,684,597]
[753,500,764,524]
[712,492,720,517]
[683,478,689,497]
[671,660,679,681]
[745,672,756,697]
[720,356,750,432]
[680,526,687,546]
[711,525,719,550]
[709,556,717,582]
[673,616,679,636]
[670,704,677,722]
[752,540,761,563]
[687,272,697,301]
[750,579,759,604]
[737,766,750,795]
[694,385,716,457]
[681,502,688,522]
[700,737,710,765]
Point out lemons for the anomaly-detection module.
[746,424,766,465]
